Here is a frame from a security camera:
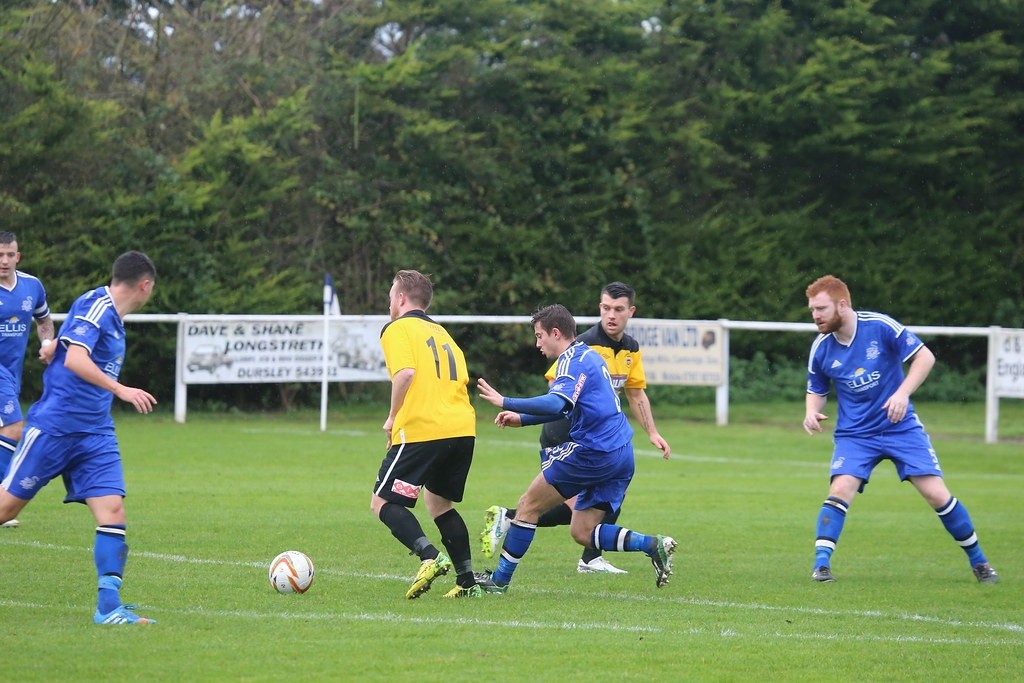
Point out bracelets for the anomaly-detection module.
[42,339,51,347]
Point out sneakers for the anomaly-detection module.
[405,551,453,600]
[92,603,157,626]
[811,565,834,582]
[479,505,513,560]
[442,584,482,601]
[472,566,510,596]
[645,534,678,589]
[577,555,628,575]
[971,562,999,586]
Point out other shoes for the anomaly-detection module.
[2,518,20,527]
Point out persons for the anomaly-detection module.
[479,281,671,574]
[469,302,678,595]
[0,230,55,529]
[370,270,483,599]
[0,250,162,625]
[803,274,998,584]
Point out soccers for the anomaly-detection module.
[269,551,316,595]
